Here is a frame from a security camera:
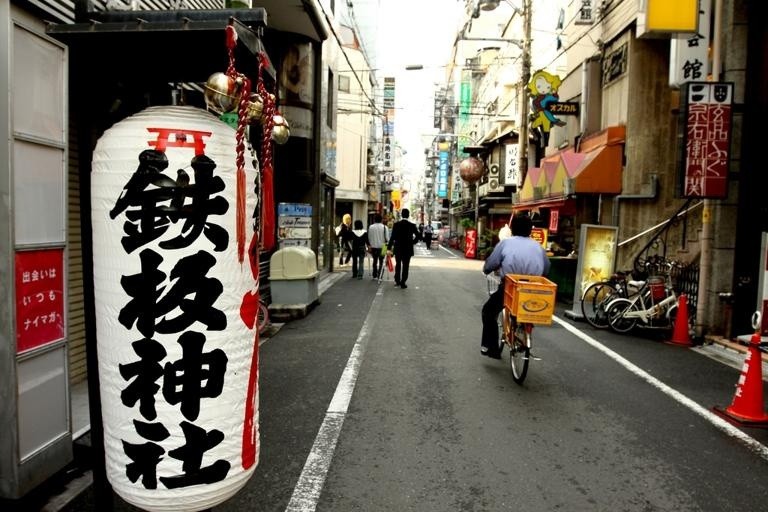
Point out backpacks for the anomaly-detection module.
[351,232,366,256]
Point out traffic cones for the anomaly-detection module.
[707,332,768,430]
[663,294,694,349]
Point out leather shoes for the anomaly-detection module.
[395,280,407,289]
[481,347,501,359]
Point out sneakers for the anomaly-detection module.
[352,275,363,280]
[373,273,377,278]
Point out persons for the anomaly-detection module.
[335,208,420,288]
[424,220,433,250]
[479,213,551,360]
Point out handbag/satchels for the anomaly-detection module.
[381,242,388,257]
[386,255,394,271]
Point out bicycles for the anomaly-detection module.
[580,253,680,334]
[483,272,543,385]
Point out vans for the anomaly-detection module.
[426,221,443,240]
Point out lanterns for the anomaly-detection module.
[91,106,261,512]
